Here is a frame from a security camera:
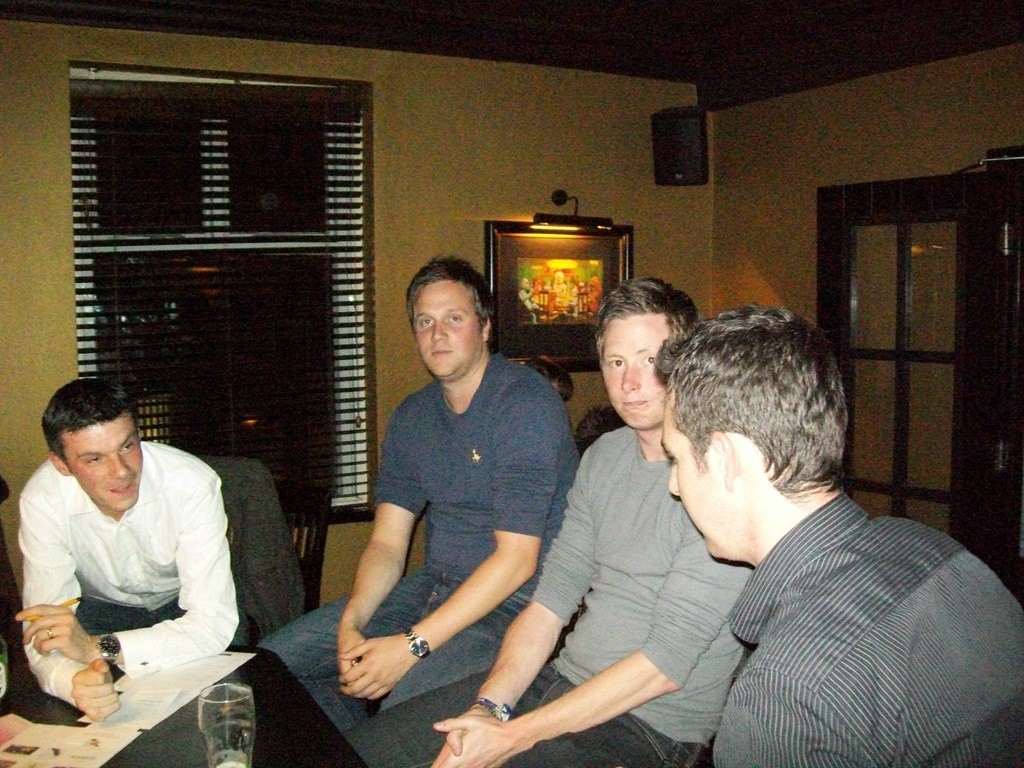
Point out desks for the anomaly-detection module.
[0,641,366,768]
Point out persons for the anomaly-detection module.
[256,252,579,732]
[341,280,758,768]
[16,377,240,722]
[653,303,1024,768]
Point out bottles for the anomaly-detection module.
[0,634,10,715]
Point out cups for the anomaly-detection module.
[196,683,256,768]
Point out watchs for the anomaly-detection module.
[401,624,431,661]
[96,634,123,665]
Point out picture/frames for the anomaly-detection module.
[481,220,635,373]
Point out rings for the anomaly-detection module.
[47,628,54,638]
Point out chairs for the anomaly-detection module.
[192,453,332,656]
